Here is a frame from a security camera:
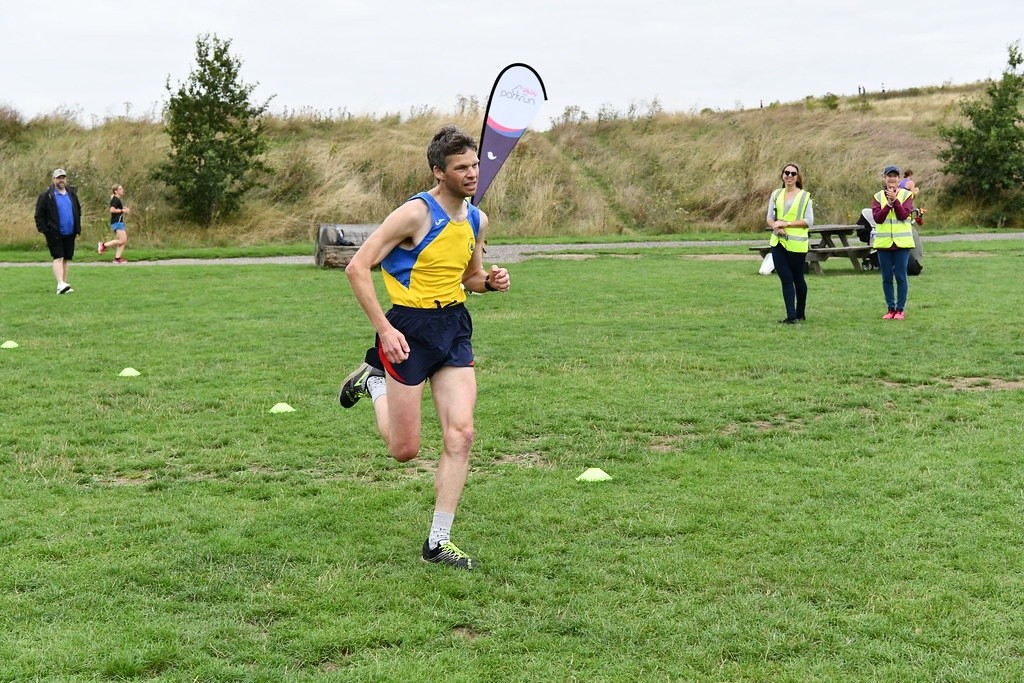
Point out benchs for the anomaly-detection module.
[316,223,381,267]
[749,244,873,261]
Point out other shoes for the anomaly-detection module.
[784,317,798,324]
[795,315,806,320]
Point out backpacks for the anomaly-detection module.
[906,253,923,276]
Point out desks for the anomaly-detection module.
[765,225,865,275]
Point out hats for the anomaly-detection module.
[884,166,900,176]
[53,169,67,178]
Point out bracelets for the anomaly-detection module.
[121,209,122,212]
[788,222,790,226]
[887,203,892,207]
[892,198,896,203]
[485,275,497,291]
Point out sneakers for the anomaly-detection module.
[338,361,385,408]
[883,309,896,319]
[57,282,75,293]
[421,537,477,571]
[112,256,128,264]
[894,310,905,319]
[98,241,105,255]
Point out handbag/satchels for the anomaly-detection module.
[759,252,775,275]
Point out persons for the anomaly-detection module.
[900,169,918,194]
[339,126,511,570]
[34,168,82,294]
[767,163,813,324]
[873,165,915,319]
[98,184,131,263]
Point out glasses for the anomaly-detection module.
[783,171,798,176]
